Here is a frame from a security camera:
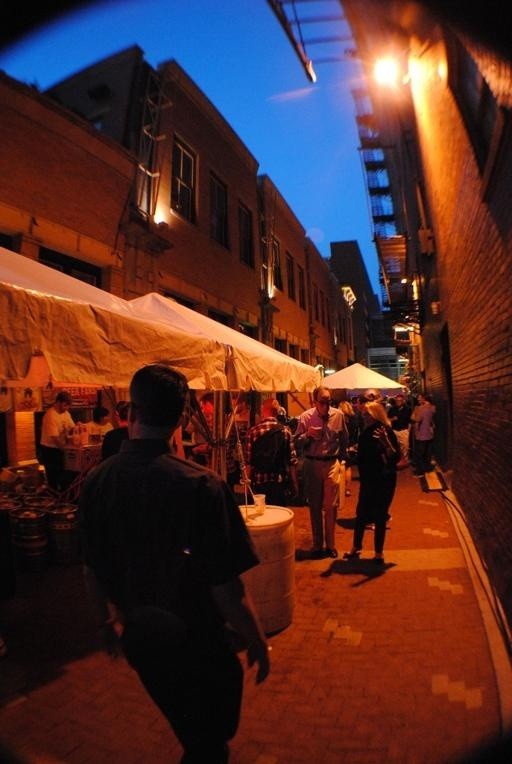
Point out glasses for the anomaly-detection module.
[318,400,332,405]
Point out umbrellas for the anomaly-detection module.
[318,359,406,400]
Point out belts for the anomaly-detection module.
[306,455,332,461]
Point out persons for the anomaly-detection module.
[88,406,114,436]
[344,400,401,565]
[294,388,349,557]
[246,398,299,507]
[102,400,129,462]
[184,410,246,486]
[72,360,270,764]
[40,391,76,490]
[363,390,436,475]
[330,394,374,496]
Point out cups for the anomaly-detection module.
[252,494,266,514]
[314,427,323,441]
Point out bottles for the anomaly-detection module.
[12,470,27,492]
[71,422,88,446]
[36,465,48,493]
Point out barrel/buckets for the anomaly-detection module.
[0,490,79,560]
[254,493,266,505]
[237,503,296,638]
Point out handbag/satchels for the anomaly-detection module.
[251,428,285,475]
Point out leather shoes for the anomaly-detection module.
[327,548,337,558]
